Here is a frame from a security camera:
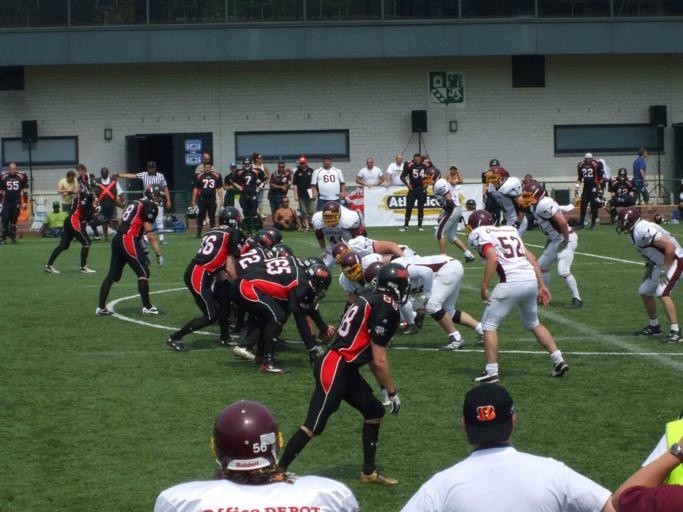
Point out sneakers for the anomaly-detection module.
[43,263,60,274]
[233,345,289,375]
[463,255,476,262]
[551,361,569,378]
[472,371,501,384]
[398,226,425,232]
[166,335,185,351]
[660,327,683,344]
[402,309,426,336]
[79,264,96,273]
[477,333,485,348]
[438,338,466,352]
[634,323,663,337]
[95,306,114,317]
[380,384,401,415]
[359,469,399,486]
[570,297,584,309]
[159,239,169,246]
[216,337,241,347]
[142,304,161,315]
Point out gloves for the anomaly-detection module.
[642,265,654,282]
[308,344,327,367]
[656,267,671,286]
[144,252,151,266]
[23,203,28,211]
[555,240,569,252]
[574,190,579,198]
[156,253,164,266]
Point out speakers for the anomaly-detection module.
[411,110,427,132]
[649,104,667,128]
[22,119,37,143]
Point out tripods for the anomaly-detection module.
[640,128,669,205]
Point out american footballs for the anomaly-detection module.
[315,325,336,344]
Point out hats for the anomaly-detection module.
[230,162,237,170]
[299,157,307,164]
[147,161,157,168]
[52,200,60,208]
[462,382,515,445]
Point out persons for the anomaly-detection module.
[191,153,346,237]
[465,210,570,382]
[653,196,682,224]
[96,183,167,316]
[356,154,475,263]
[576,150,650,229]
[482,160,583,308]
[401,383,616,512]
[0,163,29,243]
[277,263,412,484]
[616,208,683,342]
[641,419,683,485]
[612,438,683,512]
[167,205,335,374]
[312,204,485,348]
[153,401,359,512]
[43,162,171,273]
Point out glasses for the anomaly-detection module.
[278,166,285,169]
[282,200,289,203]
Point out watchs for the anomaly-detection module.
[670,443,682,463]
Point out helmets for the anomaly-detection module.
[376,263,411,302]
[425,167,442,181]
[210,399,286,473]
[618,167,628,182]
[322,201,342,228]
[242,157,252,170]
[489,159,508,185]
[186,203,200,219]
[466,210,496,232]
[617,207,641,234]
[465,200,477,212]
[522,181,546,203]
[303,255,333,291]
[654,214,664,224]
[584,152,593,159]
[254,226,294,259]
[331,241,384,289]
[218,207,241,230]
[143,182,167,203]
